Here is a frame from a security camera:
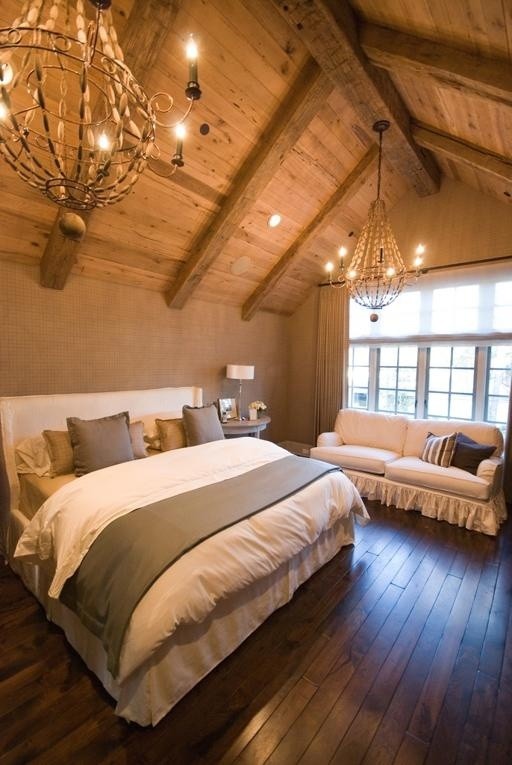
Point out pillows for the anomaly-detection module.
[182,404,225,445]
[67,411,134,476]
[419,431,457,467]
[154,417,187,451]
[42,419,148,477]
[451,431,497,475]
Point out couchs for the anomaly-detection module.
[310,408,507,535]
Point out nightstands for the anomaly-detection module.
[221,416,272,438]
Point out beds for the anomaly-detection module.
[0,385,370,727]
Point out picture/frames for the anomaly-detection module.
[219,397,237,422]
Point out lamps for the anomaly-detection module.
[2,0,202,238]
[323,120,426,322]
[226,363,254,420]
